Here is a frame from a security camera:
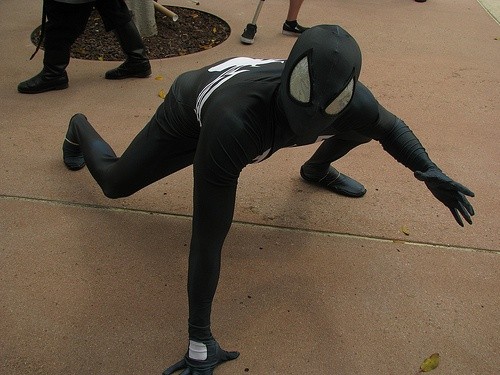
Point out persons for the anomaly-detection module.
[241,0,312,44]
[17,0,152,94]
[62,24,476,375]
